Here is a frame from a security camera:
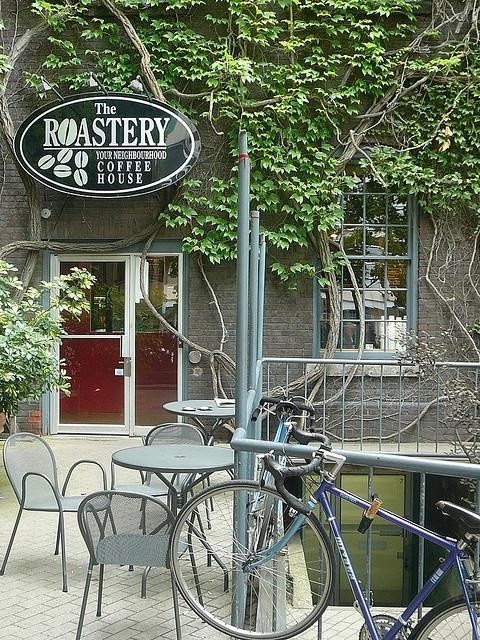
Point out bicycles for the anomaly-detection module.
[167,396,480,640]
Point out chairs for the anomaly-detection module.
[1,432,125,591]
[75,423,214,640]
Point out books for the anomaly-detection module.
[215,399,236,408]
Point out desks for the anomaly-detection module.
[162,399,236,481]
[93,444,235,599]
